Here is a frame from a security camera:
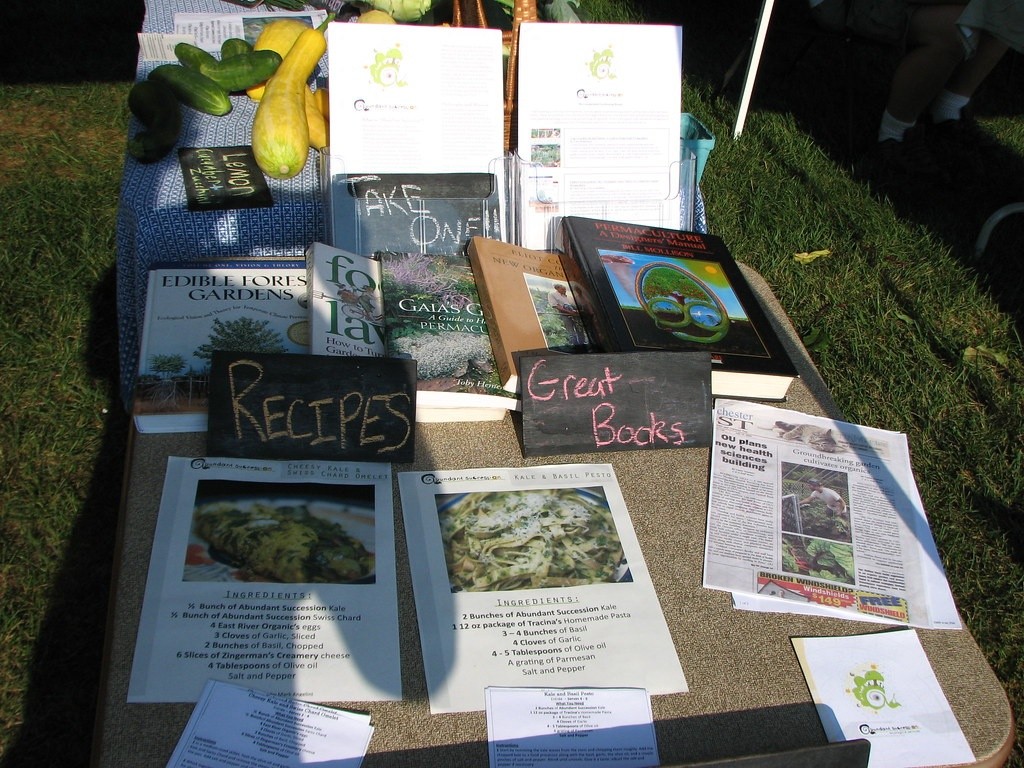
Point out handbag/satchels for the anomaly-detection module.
[680,112,716,234]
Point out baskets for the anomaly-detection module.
[449,0,539,152]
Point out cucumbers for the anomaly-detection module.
[149,37,282,117]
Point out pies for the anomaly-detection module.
[195,505,377,584]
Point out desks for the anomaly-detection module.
[96,0,1017,766]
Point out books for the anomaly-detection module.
[304,241,506,422]
[380,250,522,412]
[467,236,590,394]
[553,215,801,404]
[131,214,308,434]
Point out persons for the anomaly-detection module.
[805,0,1024,180]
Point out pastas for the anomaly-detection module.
[437,488,628,592]
